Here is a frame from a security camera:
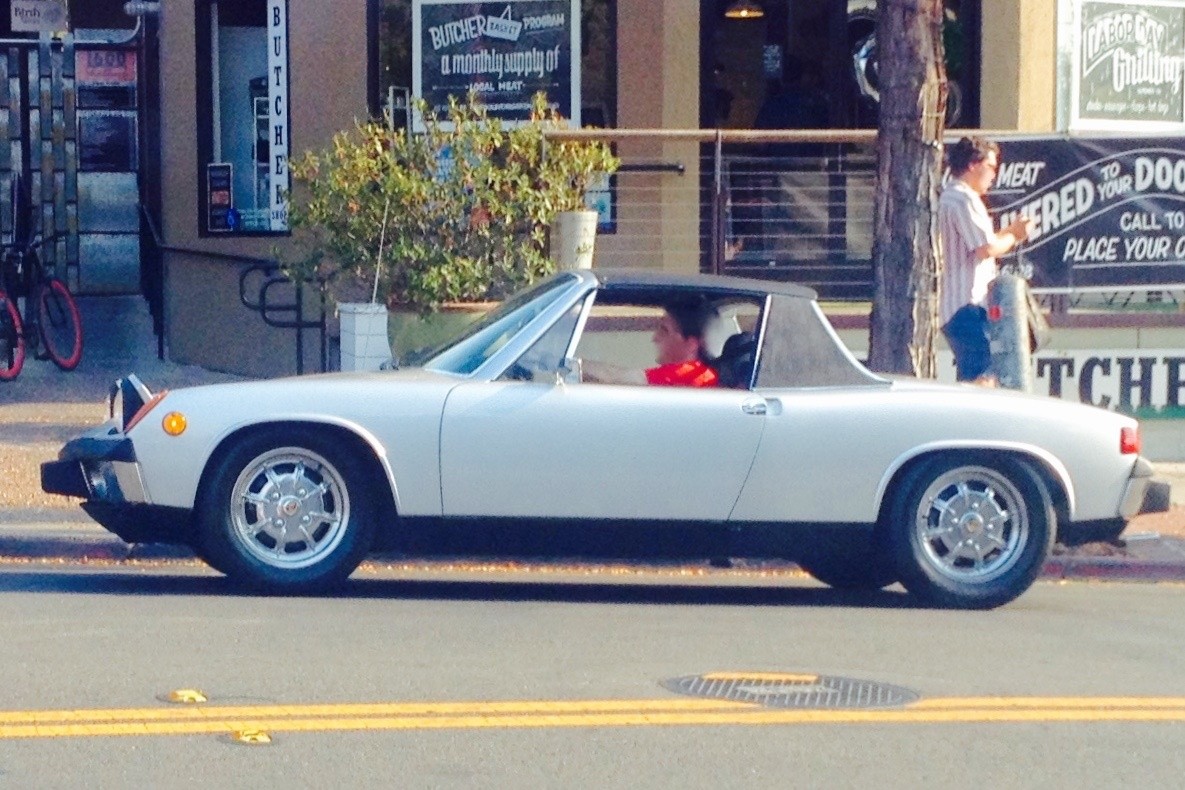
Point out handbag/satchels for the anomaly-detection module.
[1015,245,1051,352]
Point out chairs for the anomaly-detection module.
[710,330,756,391]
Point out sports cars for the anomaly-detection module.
[38,275,1175,611]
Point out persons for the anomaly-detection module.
[579,298,719,389]
[937,136,1032,382]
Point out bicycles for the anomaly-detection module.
[0,231,83,380]
